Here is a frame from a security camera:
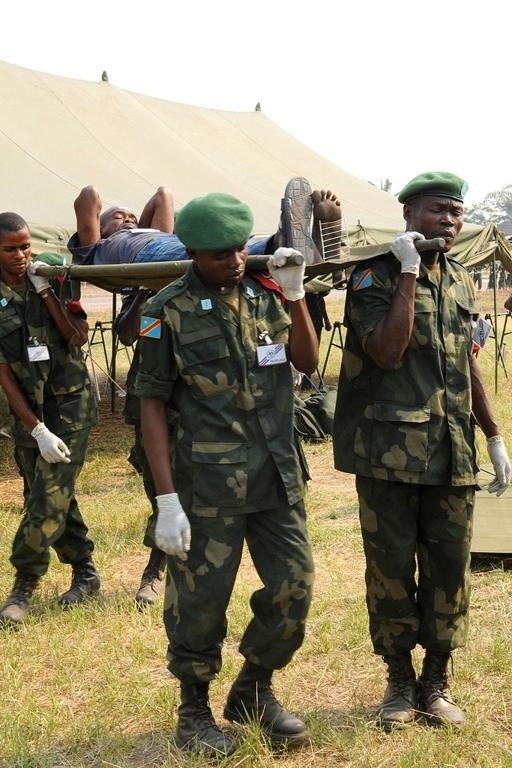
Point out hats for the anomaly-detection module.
[398,171,468,204]
[174,192,253,252]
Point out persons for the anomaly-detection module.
[0,211,101,629]
[331,169,511,730]
[297,290,332,390]
[473,260,512,291]
[65,184,343,267]
[111,288,175,613]
[130,190,321,762]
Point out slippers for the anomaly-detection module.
[277,176,314,266]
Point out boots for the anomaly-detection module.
[58,556,100,611]
[375,651,419,731]
[223,660,309,743]
[0,571,39,632]
[419,648,465,729]
[136,548,167,607]
[175,681,236,759]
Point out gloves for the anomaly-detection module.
[390,231,425,278]
[155,493,191,561]
[266,246,306,302]
[27,260,52,293]
[30,421,71,464]
[487,435,511,497]
